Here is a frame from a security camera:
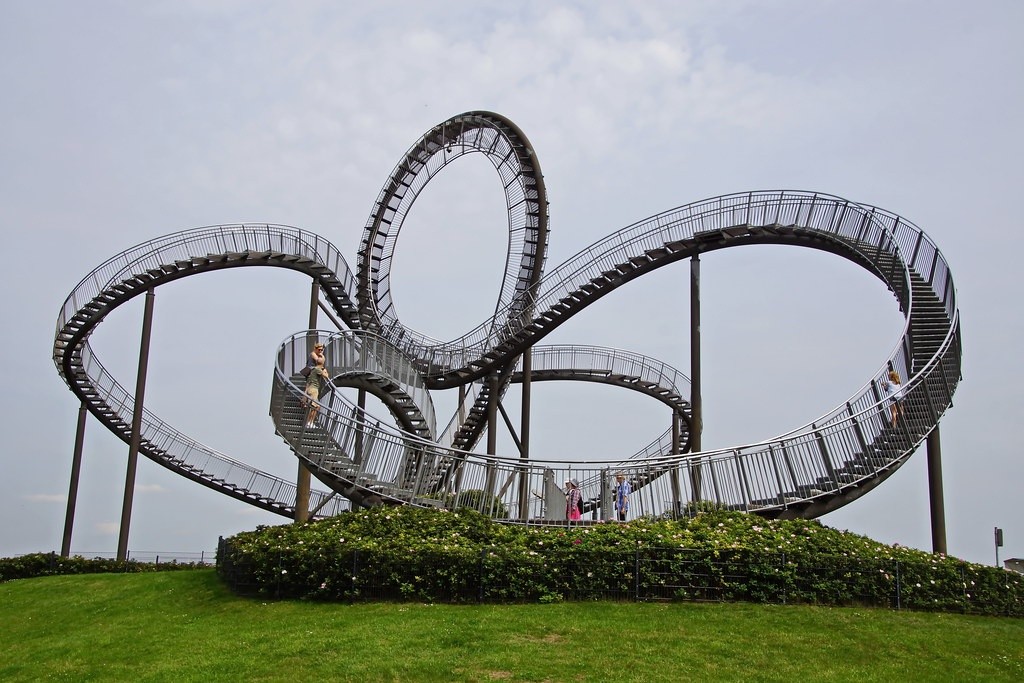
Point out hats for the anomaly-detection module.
[563,478,572,483]
[570,479,579,487]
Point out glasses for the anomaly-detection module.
[318,349,323,351]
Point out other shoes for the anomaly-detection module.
[305,421,318,429]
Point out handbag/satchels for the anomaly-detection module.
[578,494,583,510]
[300,366,311,378]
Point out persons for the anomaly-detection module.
[881,372,905,430]
[615,473,631,521]
[304,343,329,429]
[565,478,581,522]
